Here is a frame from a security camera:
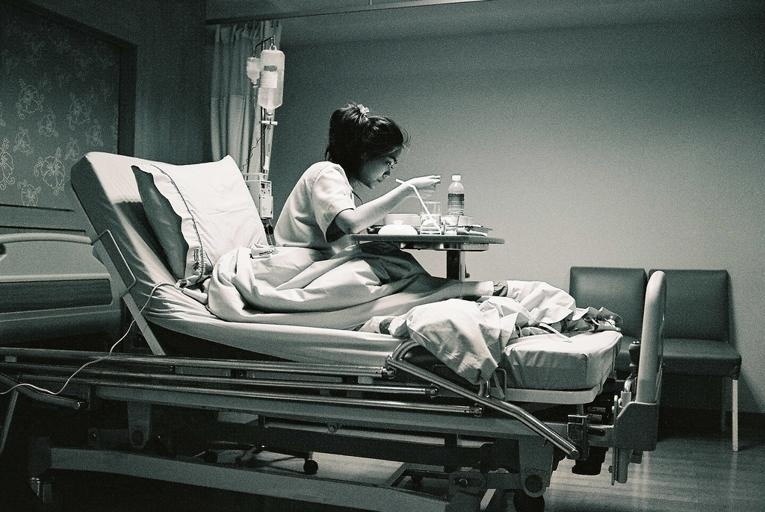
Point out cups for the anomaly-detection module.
[419,199,441,235]
[440,212,461,236]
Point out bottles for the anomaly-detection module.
[257,45,285,116]
[246,57,260,87]
[447,174,465,216]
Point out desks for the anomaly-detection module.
[350,236,506,280]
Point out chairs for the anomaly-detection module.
[569,266,646,372]
[649,270,740,452]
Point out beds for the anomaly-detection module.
[0,152,667,512]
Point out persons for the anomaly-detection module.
[274,104,442,248]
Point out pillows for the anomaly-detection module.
[131,155,271,285]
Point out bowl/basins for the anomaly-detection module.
[381,212,419,228]
